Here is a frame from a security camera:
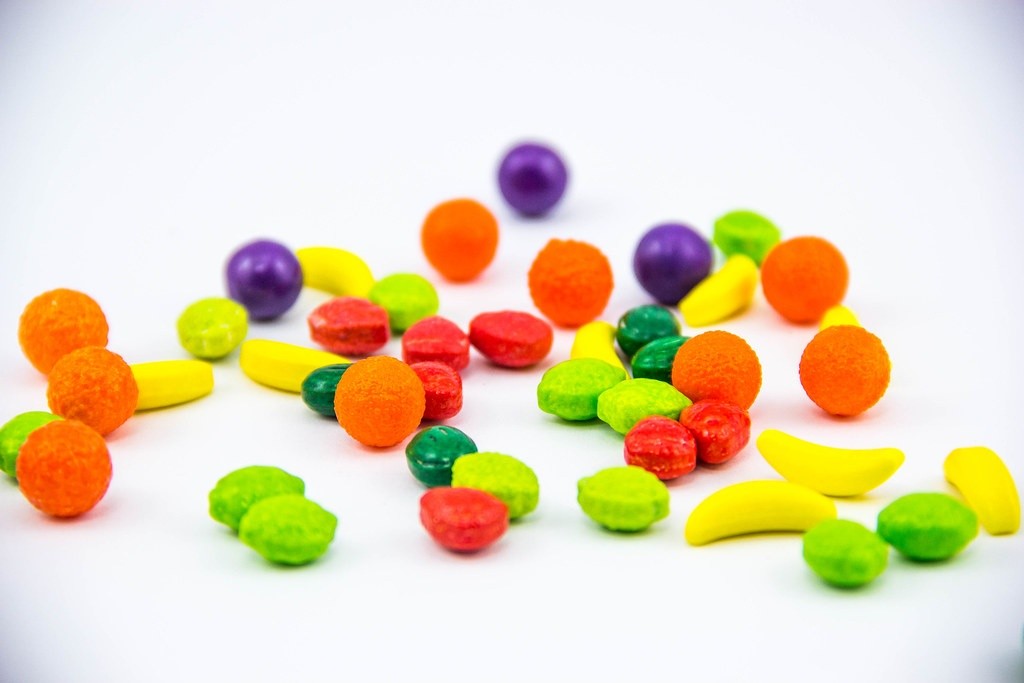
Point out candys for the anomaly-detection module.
[0,146,1018,588]
